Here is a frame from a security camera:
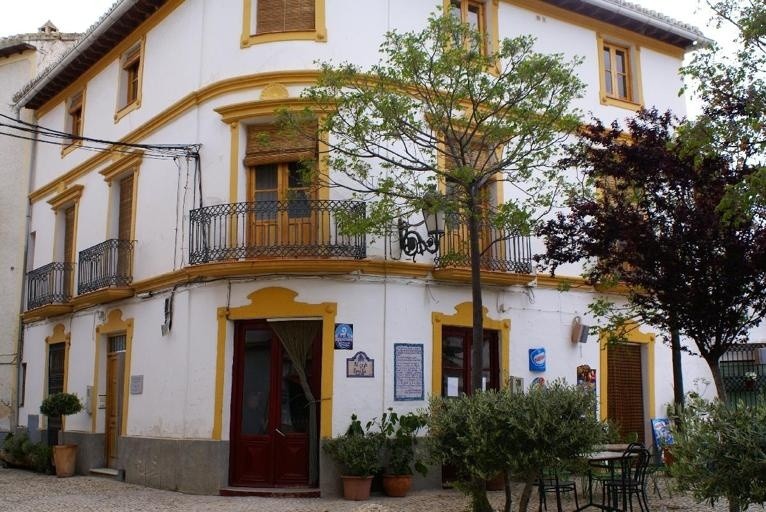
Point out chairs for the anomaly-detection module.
[540,431,664,512]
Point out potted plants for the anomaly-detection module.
[321,414,386,501]
[383,407,425,496]
[39,387,82,477]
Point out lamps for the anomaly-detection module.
[397,183,446,264]
